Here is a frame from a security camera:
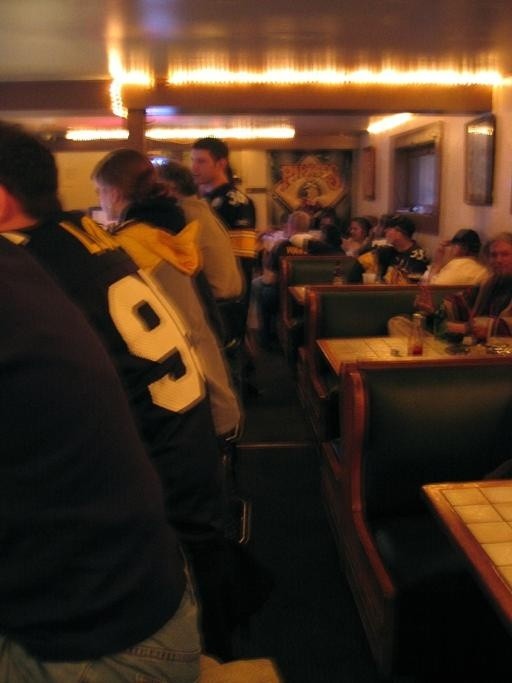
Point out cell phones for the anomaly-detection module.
[446,343,473,354]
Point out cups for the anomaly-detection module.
[473,316,494,346]
[361,272,377,284]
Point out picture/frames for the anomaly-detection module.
[463,114,496,206]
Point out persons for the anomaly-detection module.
[1,123,256,683]
[253,212,512,338]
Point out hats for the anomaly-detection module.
[440,228,481,247]
[381,215,415,237]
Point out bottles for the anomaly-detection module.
[408,312,424,357]
